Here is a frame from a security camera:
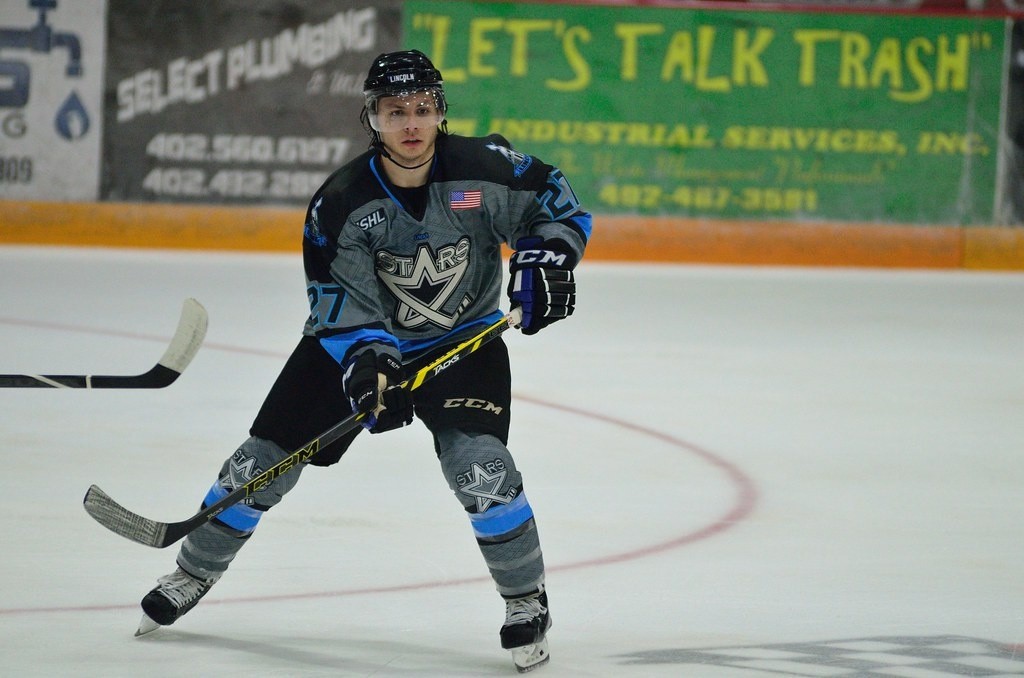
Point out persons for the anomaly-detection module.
[133,48,593,675]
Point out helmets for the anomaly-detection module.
[363,49,446,132]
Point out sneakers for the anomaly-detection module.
[134,567,216,638]
[500,584,553,673]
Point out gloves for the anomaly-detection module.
[508,235,577,336]
[340,339,414,434]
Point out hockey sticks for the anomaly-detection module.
[0,297,209,388]
[84,306,523,550]
